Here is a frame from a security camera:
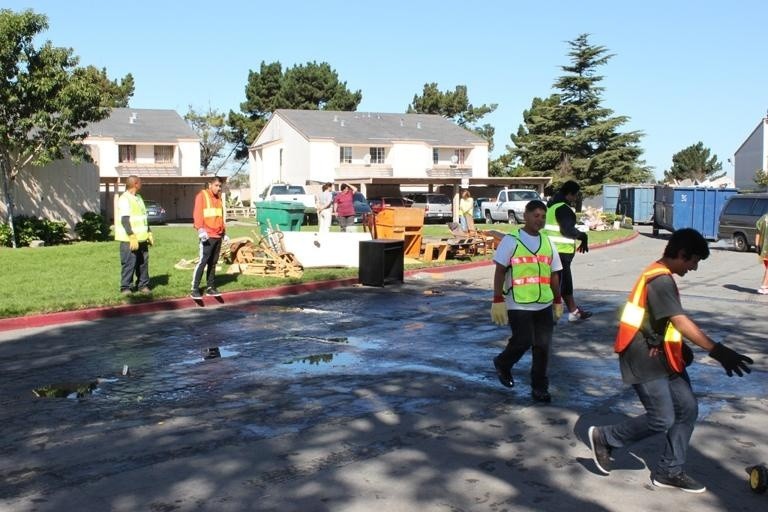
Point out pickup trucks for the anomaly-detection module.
[259,183,320,226]
[480,189,547,225]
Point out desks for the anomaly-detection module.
[372,207,425,259]
[226,206,254,218]
[422,237,494,263]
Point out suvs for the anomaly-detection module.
[404,193,452,224]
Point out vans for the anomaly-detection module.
[717,193,768,252]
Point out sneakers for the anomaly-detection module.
[190,290,202,300]
[757,285,767,293]
[569,307,592,321]
[206,287,222,296]
[653,471,706,493]
[588,426,611,474]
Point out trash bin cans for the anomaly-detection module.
[253,200,306,235]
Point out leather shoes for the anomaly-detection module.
[531,386,552,401]
[493,357,514,387]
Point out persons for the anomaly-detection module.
[755,213,768,295]
[189,176,226,301]
[113,173,155,295]
[332,181,357,233]
[586,227,755,493]
[490,199,566,402]
[457,189,475,232]
[315,182,334,232]
[543,180,593,323]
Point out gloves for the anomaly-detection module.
[128,234,140,252]
[577,240,588,254]
[491,301,509,326]
[147,232,154,246]
[708,342,753,377]
[552,303,563,321]
[198,228,209,242]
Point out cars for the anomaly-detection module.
[366,196,412,217]
[143,199,167,225]
[330,191,372,226]
[472,197,492,224]
